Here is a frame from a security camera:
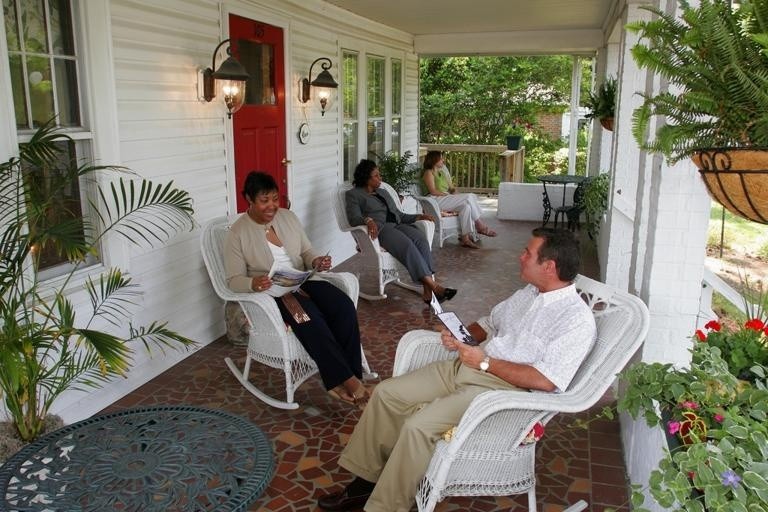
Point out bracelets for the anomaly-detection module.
[364,217,372,224]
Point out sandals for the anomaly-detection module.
[477,226,496,237]
[460,239,480,249]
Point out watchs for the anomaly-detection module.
[480,357,491,375]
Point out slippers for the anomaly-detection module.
[327,387,354,405]
[352,382,371,410]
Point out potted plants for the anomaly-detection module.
[505,127,526,150]
[368,149,424,205]
[0,110,202,512]
[624,0,767,224]
[583,75,617,131]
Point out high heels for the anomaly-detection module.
[439,288,457,301]
[425,296,448,313]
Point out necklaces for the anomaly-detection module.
[265,228,270,234]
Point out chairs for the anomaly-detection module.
[405,162,478,248]
[199,212,379,410]
[553,184,586,232]
[389,273,652,511]
[332,180,453,301]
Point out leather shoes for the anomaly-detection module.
[318,487,371,511]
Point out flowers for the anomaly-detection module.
[666,419,681,435]
[680,399,700,414]
[744,318,767,336]
[712,412,727,422]
[695,319,723,341]
[720,468,741,488]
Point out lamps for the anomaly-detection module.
[298,56,338,118]
[197,38,251,119]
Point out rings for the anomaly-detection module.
[370,230,373,233]
[258,286,262,290]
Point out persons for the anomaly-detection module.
[224,171,371,413]
[420,151,497,249]
[315,228,598,512]
[345,159,458,306]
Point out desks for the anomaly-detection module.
[1,404,274,512]
[535,174,587,227]
